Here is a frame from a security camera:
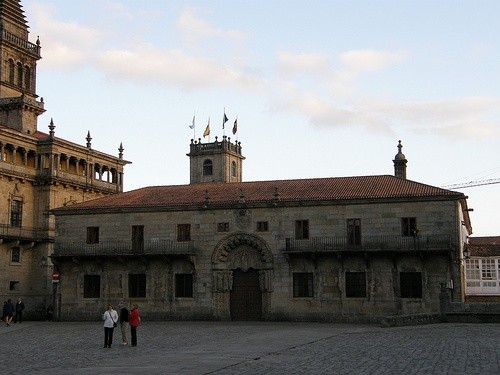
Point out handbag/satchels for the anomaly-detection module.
[113,322,117,328]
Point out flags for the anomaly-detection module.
[203,125,210,137]
[232,120,239,134]
[190,115,196,128]
[222,112,228,129]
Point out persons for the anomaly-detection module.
[0,301,7,322]
[14,298,25,323]
[128,304,143,347]
[4,298,15,327]
[118,304,130,345]
[101,304,119,348]
[47,304,54,322]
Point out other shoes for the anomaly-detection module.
[103,345,107,348]
[108,345,111,348]
[122,342,128,345]
[6,324,10,327]
